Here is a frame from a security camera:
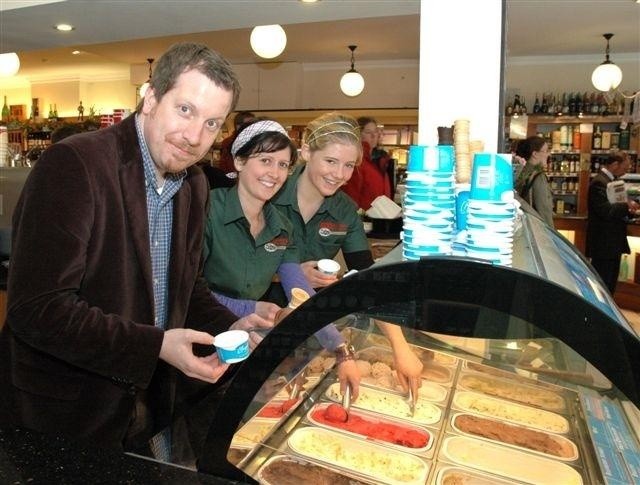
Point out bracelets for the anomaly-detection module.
[333,343,354,362]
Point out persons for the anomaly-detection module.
[510,136,555,227]
[370,123,399,199]
[345,118,391,210]
[0,43,274,452]
[201,117,361,405]
[260,113,423,403]
[585,151,639,293]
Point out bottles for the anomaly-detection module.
[555,92,562,114]
[2,96,9,125]
[576,91,584,114]
[584,92,591,113]
[570,157,575,173]
[520,96,527,114]
[569,91,577,115]
[591,94,599,112]
[594,125,602,149]
[568,177,574,191]
[575,157,581,172]
[599,94,607,113]
[533,94,541,113]
[544,157,570,173]
[548,92,554,114]
[541,92,548,114]
[562,93,569,114]
[512,94,521,115]
[561,176,568,191]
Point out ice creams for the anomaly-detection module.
[420,382,444,401]
[462,374,565,411]
[353,359,404,392]
[264,462,361,485]
[353,387,435,423]
[441,472,496,485]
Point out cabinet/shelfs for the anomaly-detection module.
[505,114,640,217]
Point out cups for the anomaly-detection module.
[316,259,341,277]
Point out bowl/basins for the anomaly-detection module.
[214,330,251,365]
[401,144,457,262]
[464,153,517,270]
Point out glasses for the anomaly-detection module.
[539,149,549,153]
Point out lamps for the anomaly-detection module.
[338,68,364,98]
[139,82,150,100]
[0,51,22,76]
[590,60,622,92]
[248,24,289,60]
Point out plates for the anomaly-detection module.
[461,361,564,391]
[325,383,442,425]
[457,373,568,414]
[258,454,373,485]
[358,378,448,403]
[355,345,453,383]
[437,467,521,485]
[366,333,459,369]
